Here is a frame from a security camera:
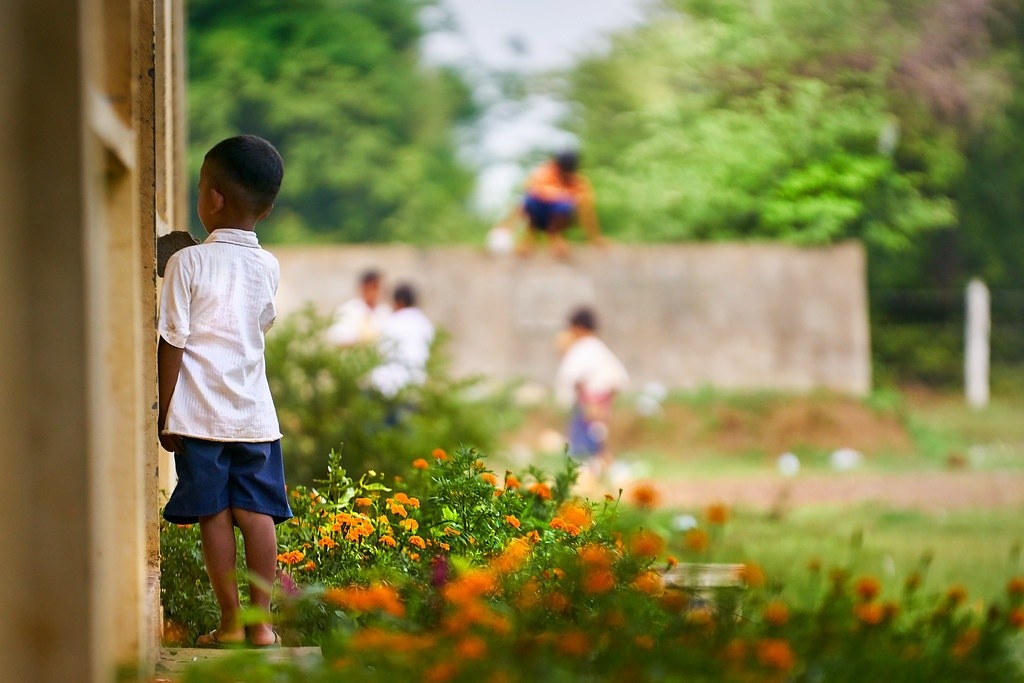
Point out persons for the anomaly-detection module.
[330,150,632,494]
[156,137,295,646]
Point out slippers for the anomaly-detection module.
[196,630,246,650]
[251,630,286,649]
